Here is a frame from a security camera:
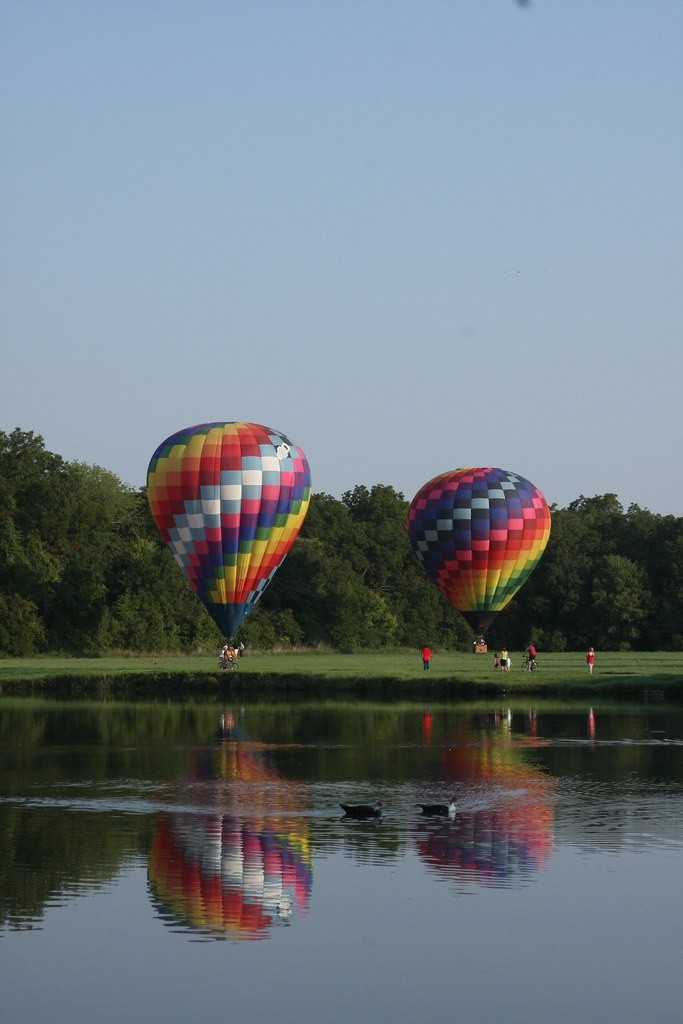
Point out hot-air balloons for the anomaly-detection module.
[145,421,312,659]
[147,704,313,946]
[407,469,551,655]
[415,707,556,889]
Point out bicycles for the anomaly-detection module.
[216,654,238,670]
[520,655,540,672]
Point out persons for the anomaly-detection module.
[238,641,245,657]
[586,647,595,675]
[219,645,236,669]
[494,647,513,672]
[479,639,485,644]
[522,642,536,672]
[473,638,477,645]
[420,644,432,671]
